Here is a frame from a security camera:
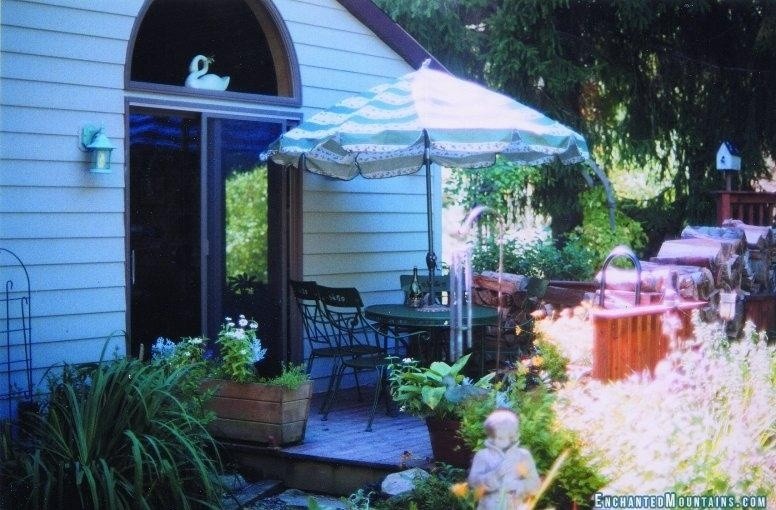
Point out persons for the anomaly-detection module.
[469,409,540,510]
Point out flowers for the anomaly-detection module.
[150,313,263,384]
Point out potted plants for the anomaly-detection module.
[389,357,501,475]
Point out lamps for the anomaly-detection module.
[79,125,117,174]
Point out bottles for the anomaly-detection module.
[406,268,424,309]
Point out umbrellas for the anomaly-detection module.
[258,57,590,308]
[127,112,283,153]
